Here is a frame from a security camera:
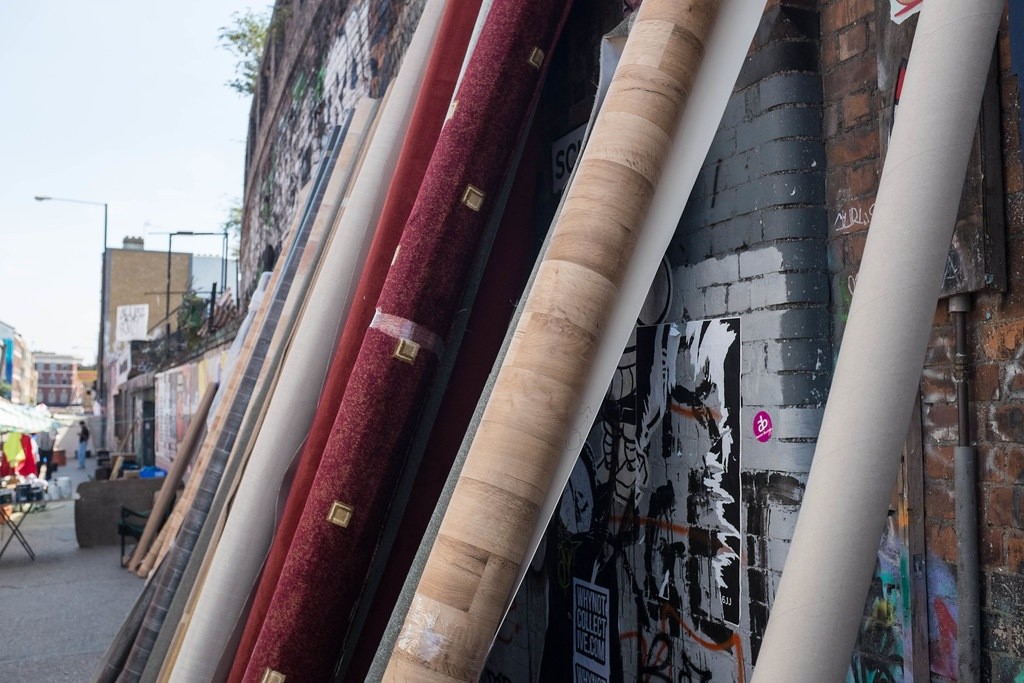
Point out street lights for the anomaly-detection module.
[36,192,109,408]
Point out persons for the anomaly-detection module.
[35,430,60,481]
[77,420,89,469]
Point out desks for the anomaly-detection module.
[0,498,48,561]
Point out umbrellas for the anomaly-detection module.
[0,396,59,434]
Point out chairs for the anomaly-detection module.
[118,488,177,563]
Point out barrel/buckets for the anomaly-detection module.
[57,476,72,498]
[47,485,60,500]
